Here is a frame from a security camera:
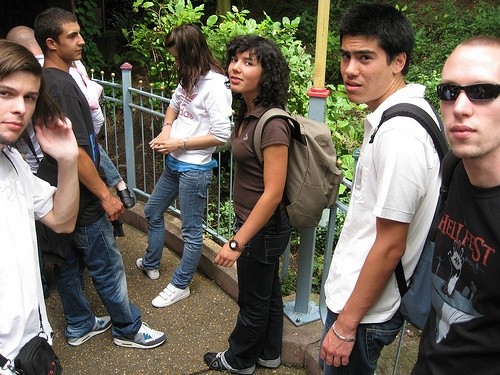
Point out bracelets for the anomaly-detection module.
[181,138,187,149]
[163,122,172,127]
[331,321,356,342]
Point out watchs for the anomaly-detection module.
[228,236,248,252]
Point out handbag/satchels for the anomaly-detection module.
[36,156,94,225]
[14,336,63,374]
[74,68,104,111]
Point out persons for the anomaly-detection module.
[0,8,169,375]
[409,35,500,375]
[200,35,292,375]
[319,0,448,375]
[136,23,236,308]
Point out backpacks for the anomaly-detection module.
[253,108,343,231]
[369,98,450,333]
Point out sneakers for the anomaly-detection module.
[67,315,112,347]
[203,352,255,375]
[114,321,166,349]
[151,282,190,308]
[136,257,159,279]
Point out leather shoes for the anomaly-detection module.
[116,185,135,208]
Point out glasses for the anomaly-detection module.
[437,82,500,102]
